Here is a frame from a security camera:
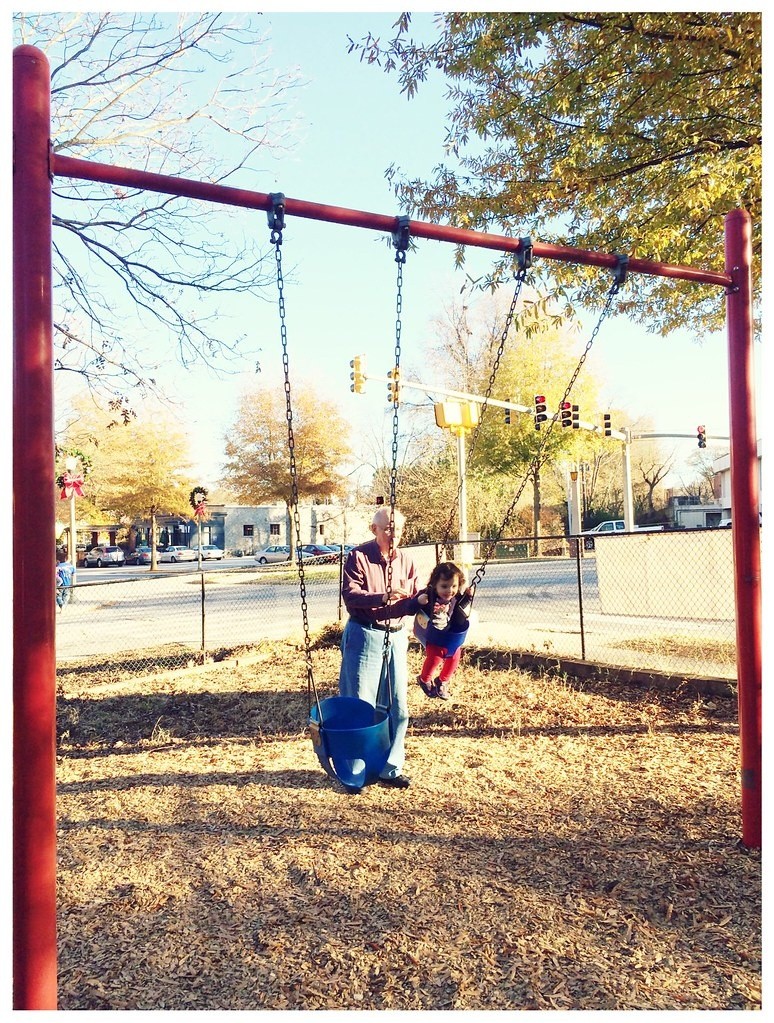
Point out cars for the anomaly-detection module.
[327,544,358,561]
[160,546,196,563]
[61,543,86,552]
[192,545,225,561]
[296,544,338,564]
[254,546,313,564]
[124,547,162,566]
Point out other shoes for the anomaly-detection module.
[379,774,410,788]
[346,785,362,794]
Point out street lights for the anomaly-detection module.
[67,456,80,604]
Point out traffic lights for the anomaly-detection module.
[570,405,579,430]
[376,496,386,504]
[505,393,519,424]
[348,354,368,393]
[534,396,547,422]
[698,425,706,448]
[602,412,611,438]
[559,402,571,428]
[388,367,399,403]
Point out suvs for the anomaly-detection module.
[83,546,125,568]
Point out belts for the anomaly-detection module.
[350,615,402,633]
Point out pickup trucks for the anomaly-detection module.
[581,520,664,549]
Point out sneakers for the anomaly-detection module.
[434,676,450,700]
[417,674,438,698]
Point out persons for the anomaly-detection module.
[409,563,474,698]
[56,554,74,613]
[335,507,422,796]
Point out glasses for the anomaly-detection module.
[384,528,407,535]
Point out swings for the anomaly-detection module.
[413,236,629,668]
[266,190,411,790]
